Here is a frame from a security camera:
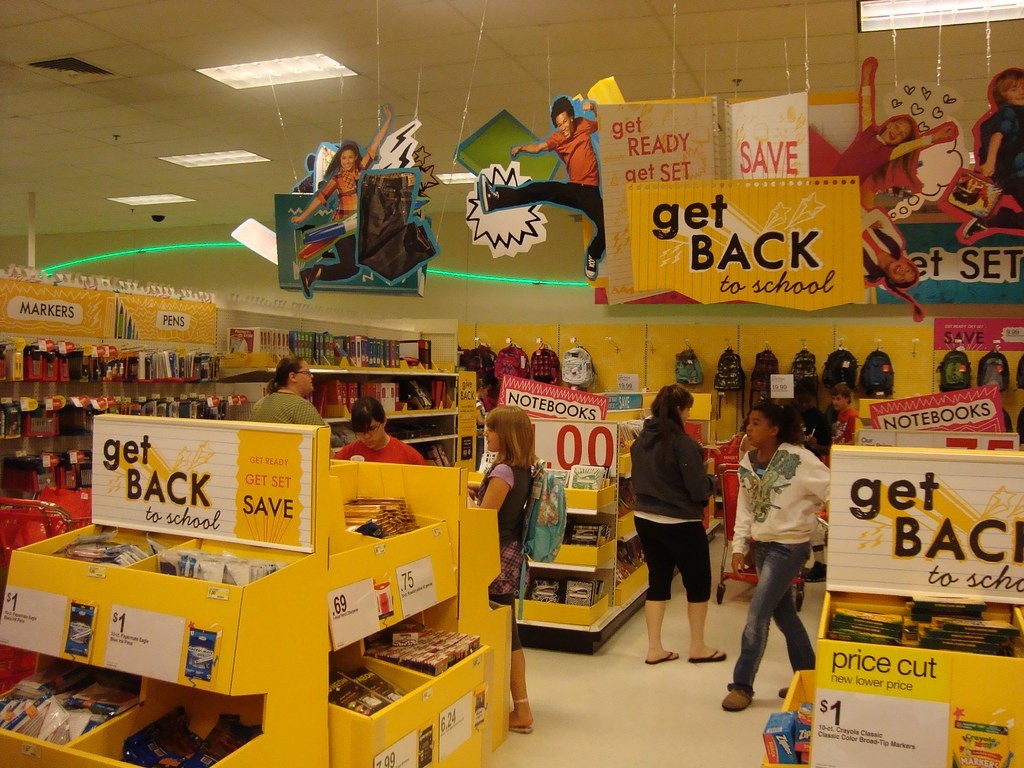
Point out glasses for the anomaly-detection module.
[367,423,380,434]
[295,370,312,377]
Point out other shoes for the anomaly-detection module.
[300,268,313,299]
[723,688,753,710]
[779,686,790,698]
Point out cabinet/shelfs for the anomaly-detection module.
[0,414,512,768]
[0,376,222,486]
[467,392,714,655]
[216,350,459,469]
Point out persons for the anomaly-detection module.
[330,397,429,465]
[738,379,859,583]
[482,97,606,281]
[835,60,953,187]
[476,378,489,472]
[467,405,534,733]
[962,67,1024,239]
[721,402,831,712]
[291,104,392,298]
[249,357,326,425]
[861,209,922,316]
[629,384,727,665]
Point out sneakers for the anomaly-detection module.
[586,254,598,276]
[803,561,827,582]
[481,176,499,212]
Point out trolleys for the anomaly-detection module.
[715,444,828,612]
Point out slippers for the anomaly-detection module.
[509,726,534,734]
[646,651,679,665]
[688,650,727,663]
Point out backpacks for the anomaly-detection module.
[791,350,815,380]
[938,349,971,391]
[530,344,560,383]
[823,350,857,388]
[674,349,704,385]
[750,350,780,391]
[520,458,568,562]
[977,350,1009,391]
[859,349,894,392]
[714,346,745,391]
[562,347,593,387]
[494,342,529,380]
